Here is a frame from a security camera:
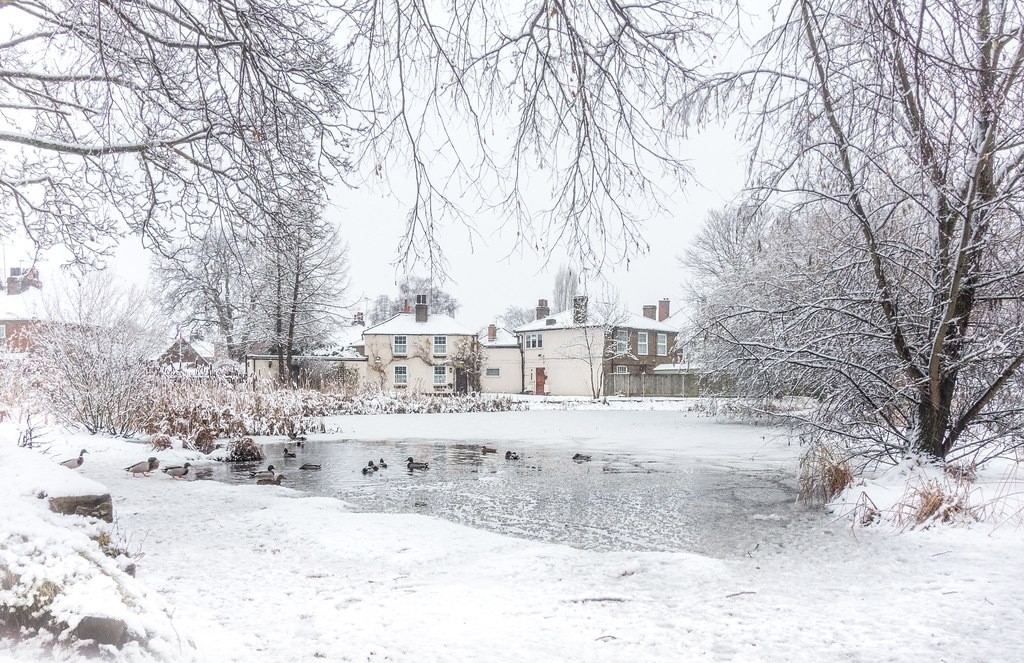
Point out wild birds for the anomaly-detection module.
[162,462,191,478]
[573,453,589,460]
[250,465,275,476]
[124,458,159,477]
[284,430,322,470]
[60,449,89,469]
[362,458,387,473]
[405,457,428,469]
[256,475,287,485]
[482,446,519,460]
